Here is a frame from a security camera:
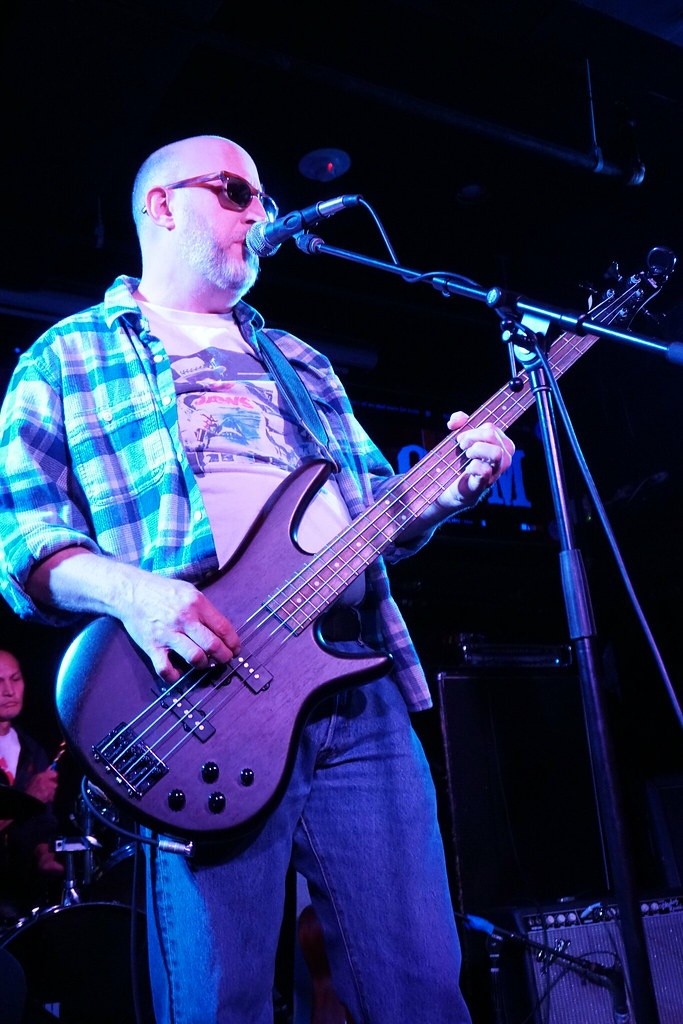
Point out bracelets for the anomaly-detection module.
[434,502,449,513]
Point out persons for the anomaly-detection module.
[0,650,79,1024]
[0,136,515,1024]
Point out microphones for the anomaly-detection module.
[612,957,630,1024]
[246,194,363,257]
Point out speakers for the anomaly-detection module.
[644,775,683,895]
[509,895,683,1024]
[437,671,613,919]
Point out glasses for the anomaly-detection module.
[142,172,279,221]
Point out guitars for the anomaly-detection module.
[53,244,678,831]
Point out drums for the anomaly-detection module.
[0,903,158,1024]
